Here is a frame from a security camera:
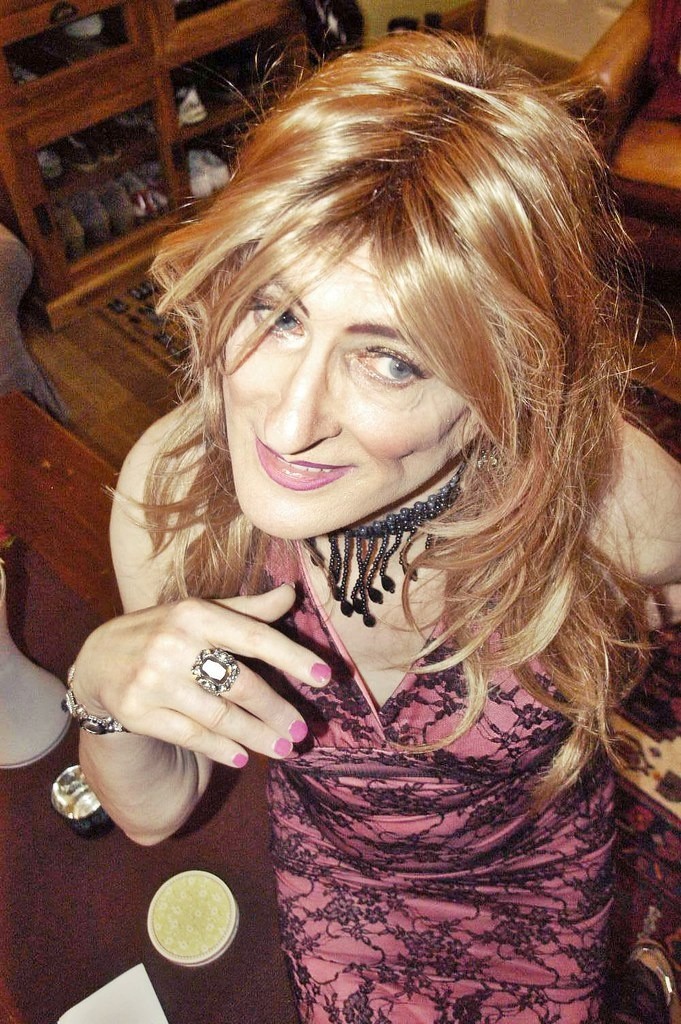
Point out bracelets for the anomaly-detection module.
[60,663,131,735]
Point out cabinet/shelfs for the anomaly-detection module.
[0,1,326,331]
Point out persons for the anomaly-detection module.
[62,29,681,1024]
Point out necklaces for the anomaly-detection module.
[300,442,474,628]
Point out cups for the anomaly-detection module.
[50,764,116,841]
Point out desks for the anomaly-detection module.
[1,385,308,1024]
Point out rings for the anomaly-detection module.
[191,648,237,694]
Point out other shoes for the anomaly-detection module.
[116,106,156,134]
[36,151,63,180]
[174,87,207,125]
[189,149,230,191]
[188,167,213,199]
[120,171,169,218]
[82,127,121,162]
[52,134,101,172]
[66,12,100,38]
[71,191,109,236]
[51,201,85,251]
[99,180,133,228]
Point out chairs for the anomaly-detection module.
[567,1,681,402]
